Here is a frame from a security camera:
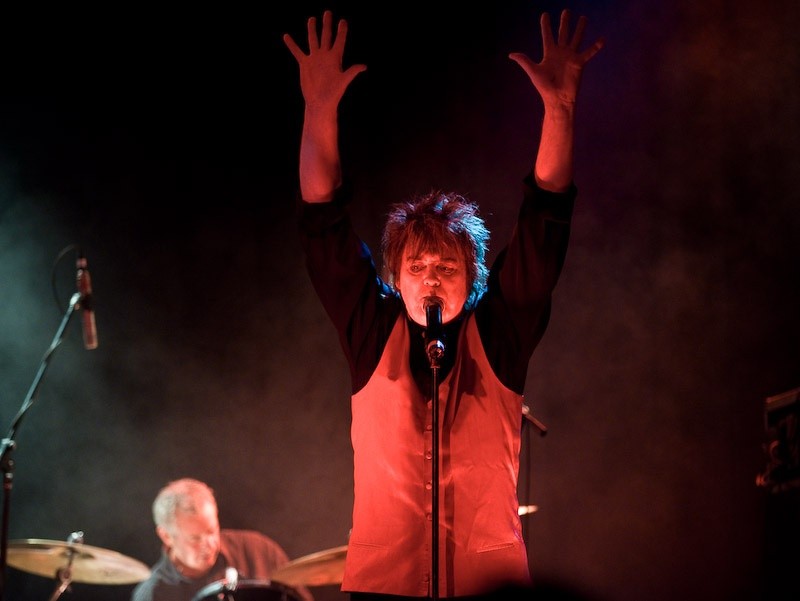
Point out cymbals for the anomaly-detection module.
[5,538,151,586]
[270,544,351,586]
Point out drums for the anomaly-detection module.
[191,575,307,601]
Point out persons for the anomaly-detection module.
[132,478,313,601]
[283,9,610,601]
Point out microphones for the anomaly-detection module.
[422,297,446,368]
[77,250,99,351]
